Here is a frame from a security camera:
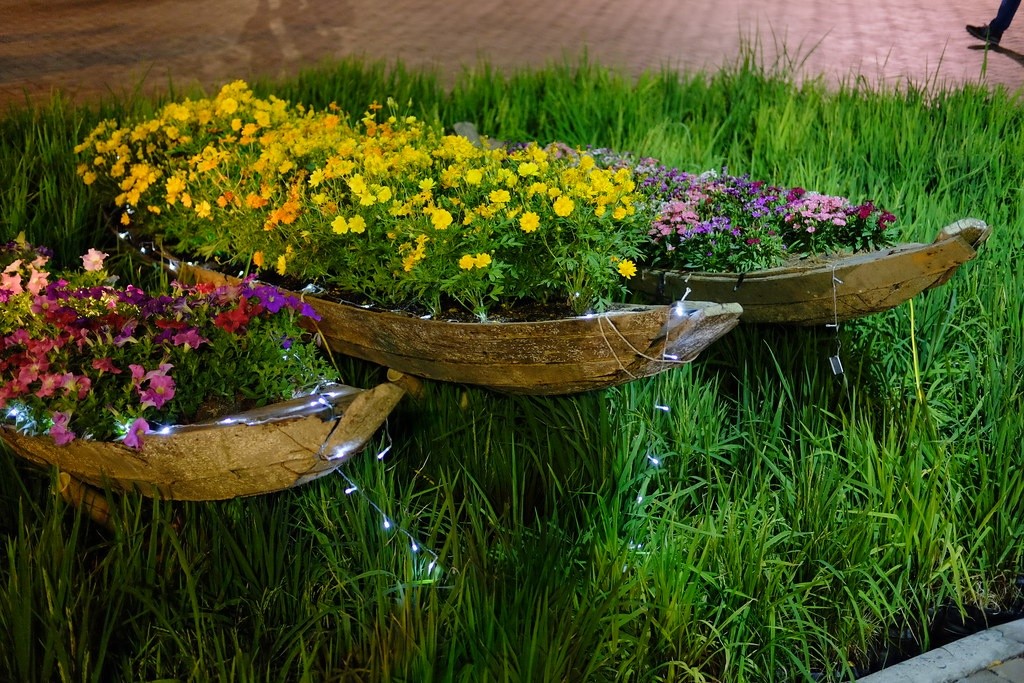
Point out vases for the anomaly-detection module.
[165,254,747,403]
[620,218,993,325]
[0,383,408,506]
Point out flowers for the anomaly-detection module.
[0,229,343,452]
[508,137,901,275]
[69,78,654,309]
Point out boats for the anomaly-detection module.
[627,216,992,326]
[148,227,746,399]
[1,383,408,573]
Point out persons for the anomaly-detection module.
[966,0,1022,46]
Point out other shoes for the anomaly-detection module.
[966,24,1003,44]
